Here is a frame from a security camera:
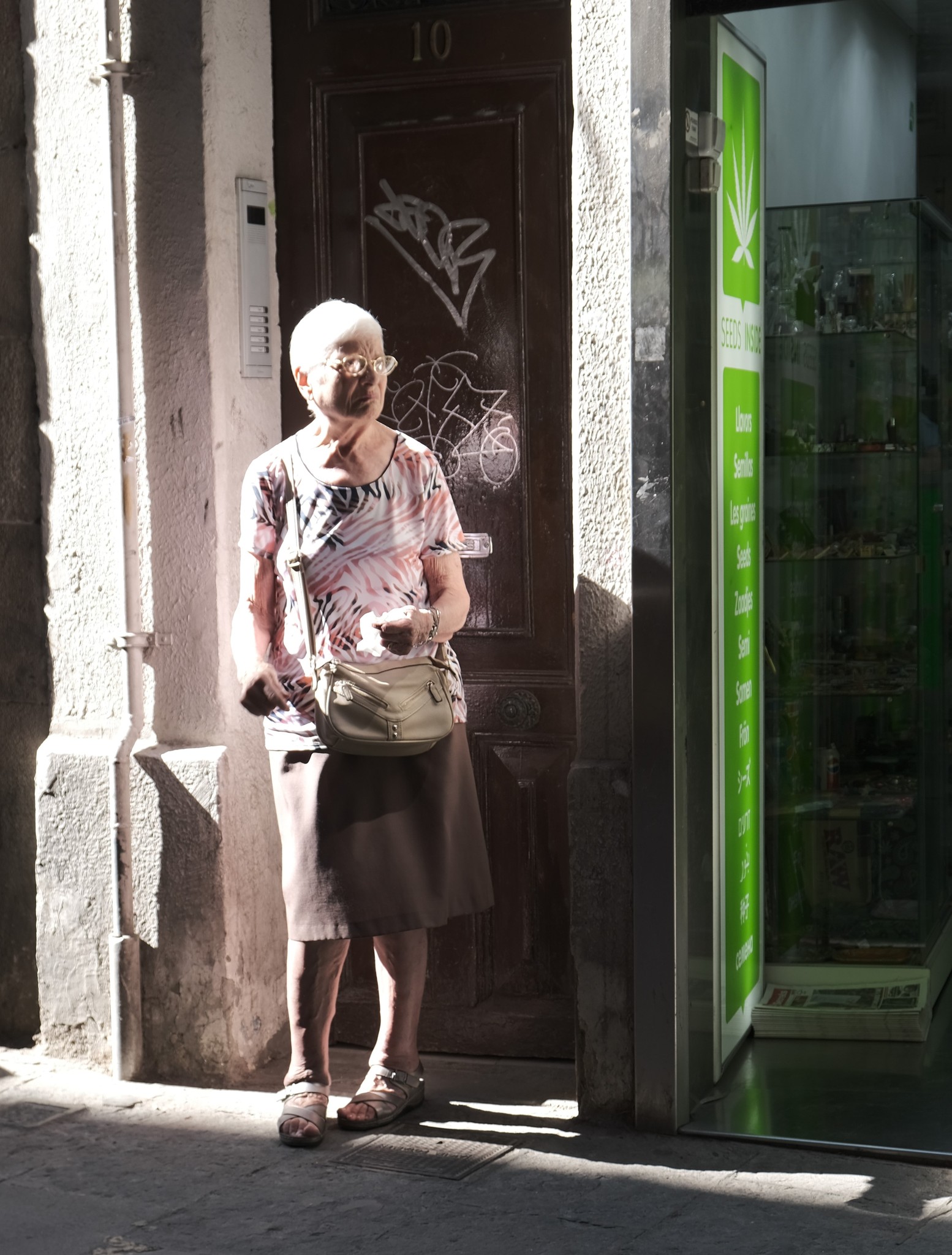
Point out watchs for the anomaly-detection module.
[413,606,440,647]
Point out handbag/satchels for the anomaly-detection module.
[308,653,455,756]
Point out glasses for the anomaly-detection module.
[322,354,399,379]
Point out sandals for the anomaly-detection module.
[337,1058,425,1130]
[274,1081,330,1147]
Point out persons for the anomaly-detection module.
[231,299,498,1147]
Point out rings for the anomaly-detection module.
[387,642,393,650]
